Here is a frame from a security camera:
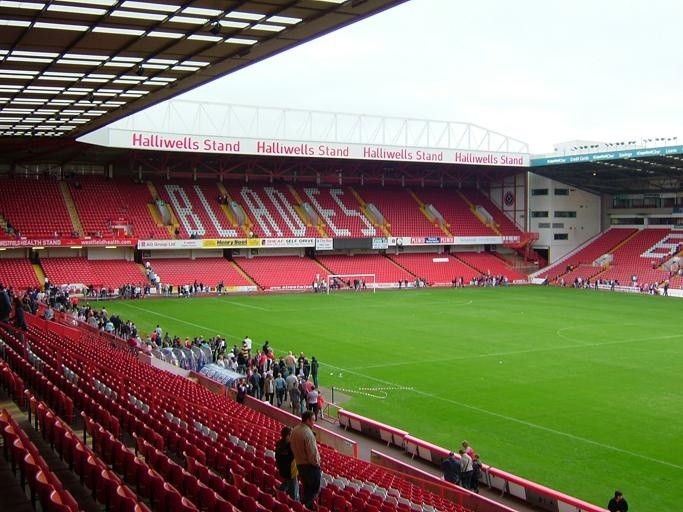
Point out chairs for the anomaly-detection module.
[0,321,468,510]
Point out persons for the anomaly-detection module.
[392,243,682,296]
[217,192,228,205]
[313,277,366,293]
[145,325,325,422]
[443,441,487,493]
[146,260,160,287]
[19,282,142,349]
[85,280,225,301]
[276,426,301,506]
[607,490,628,512]
[291,410,325,511]
[1,281,26,331]
